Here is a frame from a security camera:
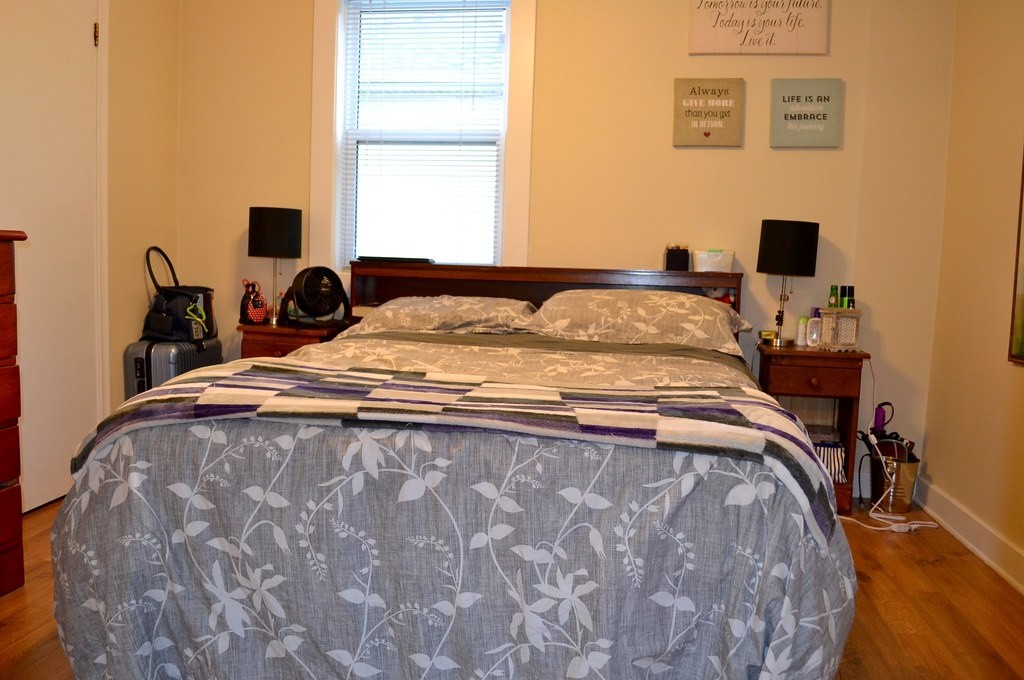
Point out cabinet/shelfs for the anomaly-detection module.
[0,229,34,595]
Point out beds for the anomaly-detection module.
[52,261,858,679]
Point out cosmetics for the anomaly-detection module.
[797,316,821,346]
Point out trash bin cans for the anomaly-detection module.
[870,456,920,513]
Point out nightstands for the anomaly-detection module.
[235,316,341,361]
[756,345,871,516]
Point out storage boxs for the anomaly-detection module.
[692,250,736,272]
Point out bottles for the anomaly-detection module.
[665,248,689,271]
[828,284,839,307]
[847,299,855,309]
[840,285,854,309]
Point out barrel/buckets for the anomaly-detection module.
[868,455,920,512]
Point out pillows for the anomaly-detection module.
[329,294,539,343]
[515,290,753,364]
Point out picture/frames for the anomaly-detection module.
[1007,166,1024,365]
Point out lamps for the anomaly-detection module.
[757,218,821,346]
[248,205,303,325]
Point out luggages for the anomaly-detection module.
[123,338,224,402]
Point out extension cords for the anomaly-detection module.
[890,523,910,532]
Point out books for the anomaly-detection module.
[357,256,435,264]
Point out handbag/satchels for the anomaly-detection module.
[240,279,271,325]
[142,246,219,343]
